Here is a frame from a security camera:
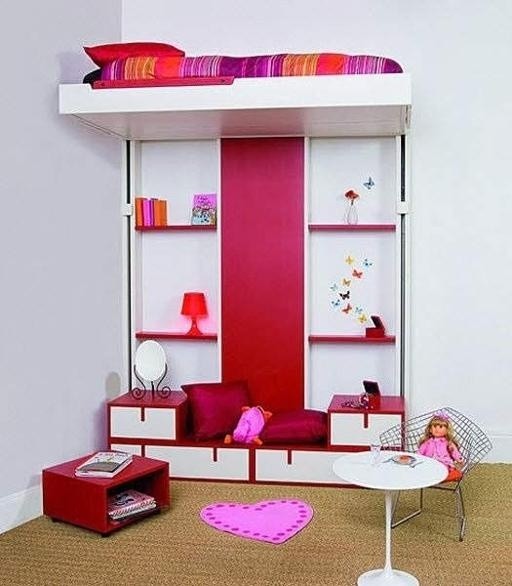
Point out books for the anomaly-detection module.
[135,194,167,226]
[75,451,133,481]
[104,487,157,523]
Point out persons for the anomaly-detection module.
[416,412,463,484]
